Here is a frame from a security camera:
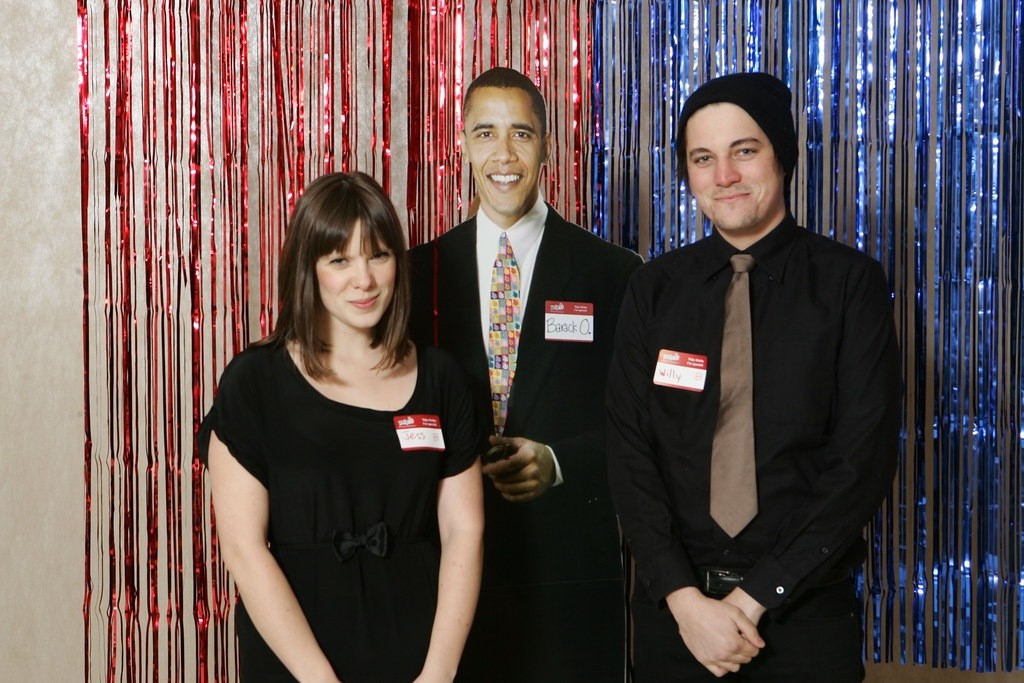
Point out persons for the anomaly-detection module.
[198,172,488,683]
[601,71,905,683]
[403,66,646,683]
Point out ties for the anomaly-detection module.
[708,254,758,540]
[486,233,520,433]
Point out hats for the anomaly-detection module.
[676,71,798,174]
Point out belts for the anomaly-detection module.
[690,565,750,596]
